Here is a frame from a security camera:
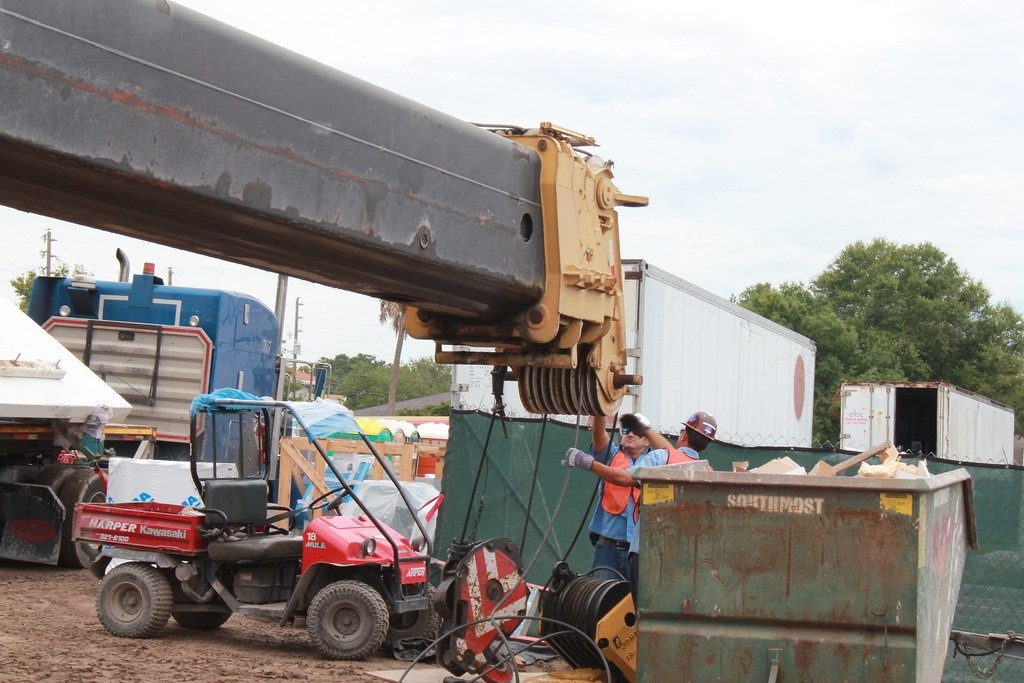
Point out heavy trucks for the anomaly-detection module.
[0,248,281,568]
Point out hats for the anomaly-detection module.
[634,413,651,428]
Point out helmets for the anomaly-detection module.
[680,411,717,441]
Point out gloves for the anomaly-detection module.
[619,413,649,438]
[561,447,595,471]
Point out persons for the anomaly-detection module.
[562,411,718,599]
[588,412,673,580]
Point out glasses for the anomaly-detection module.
[621,427,639,436]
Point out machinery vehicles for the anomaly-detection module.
[70,397,439,658]
[0,0,649,683]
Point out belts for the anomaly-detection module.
[599,535,630,551]
